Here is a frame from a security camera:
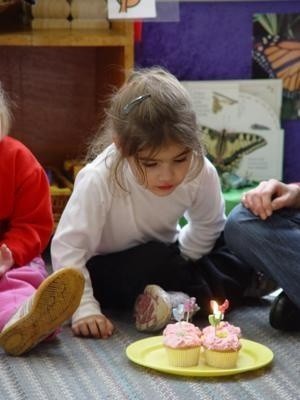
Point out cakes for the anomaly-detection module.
[162,297,202,349]
[201,299,242,351]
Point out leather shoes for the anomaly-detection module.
[269,291,300,331]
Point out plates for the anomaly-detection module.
[126,336,275,377]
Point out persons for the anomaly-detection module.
[0,87,84,354]
[224,179,300,333]
[50,66,228,338]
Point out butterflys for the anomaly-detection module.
[197,125,267,171]
[250,27,300,100]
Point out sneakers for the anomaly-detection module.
[134,284,200,332]
[0,267,85,357]
